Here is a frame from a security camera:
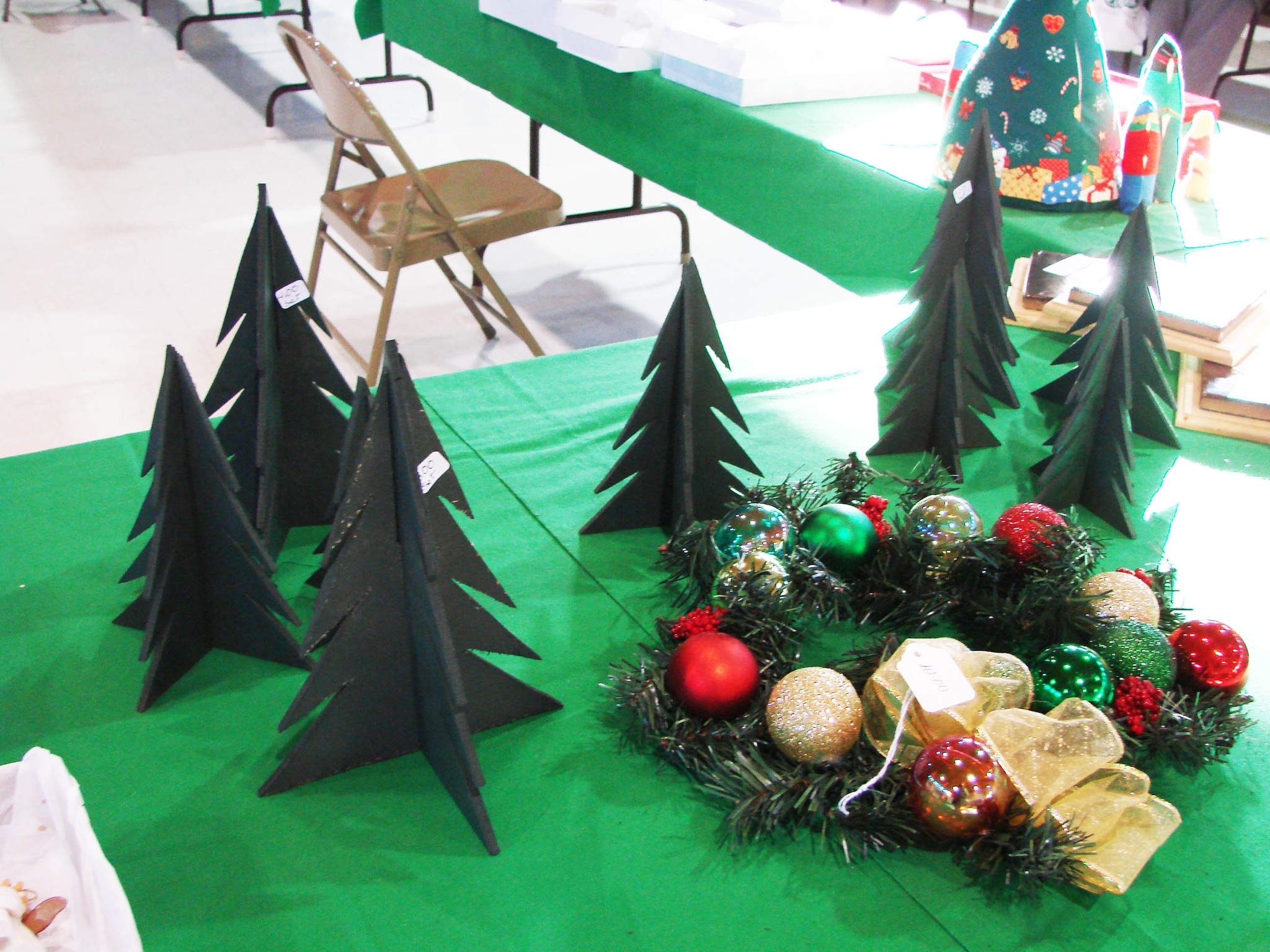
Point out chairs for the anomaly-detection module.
[278,22,568,387]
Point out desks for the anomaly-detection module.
[0,0,1270,952]
